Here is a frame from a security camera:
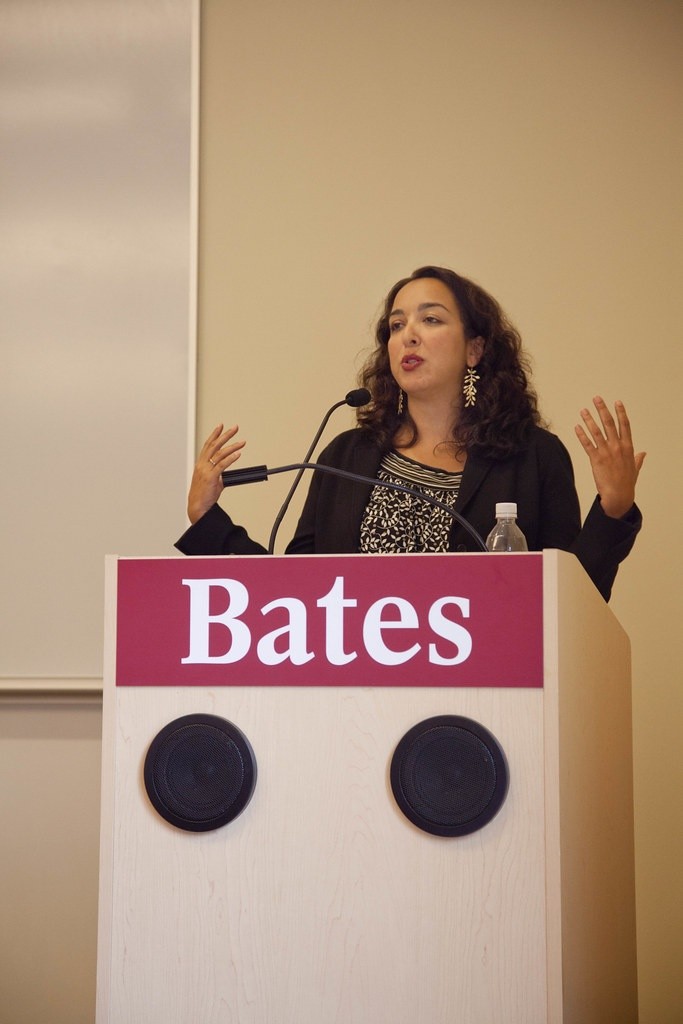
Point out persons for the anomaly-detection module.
[173,261,648,607]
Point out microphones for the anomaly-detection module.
[222,462,490,552]
[268,388,371,555]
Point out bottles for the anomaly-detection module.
[485,502,528,552]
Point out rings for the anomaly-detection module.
[210,460,216,465]
